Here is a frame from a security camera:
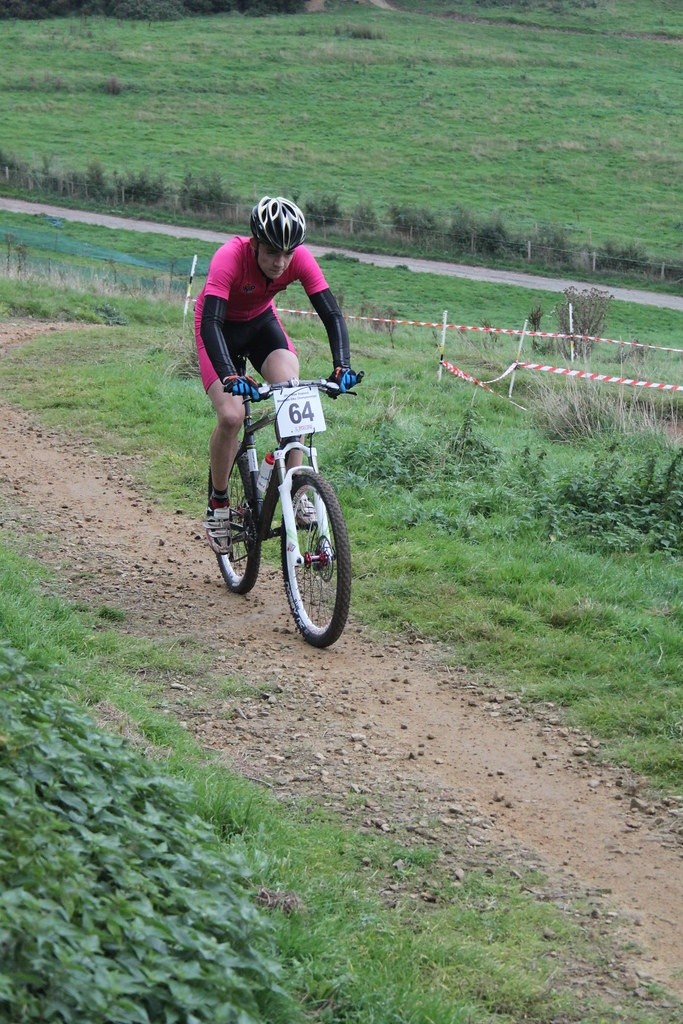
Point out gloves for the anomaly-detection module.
[327,367,358,399]
[225,375,261,403]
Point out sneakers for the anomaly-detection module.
[203,495,233,555]
[293,493,318,529]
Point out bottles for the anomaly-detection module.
[257,452,274,493]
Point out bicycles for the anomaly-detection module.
[202,371,364,648]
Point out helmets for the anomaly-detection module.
[250,196,308,252]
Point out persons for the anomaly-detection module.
[194,196,362,556]
